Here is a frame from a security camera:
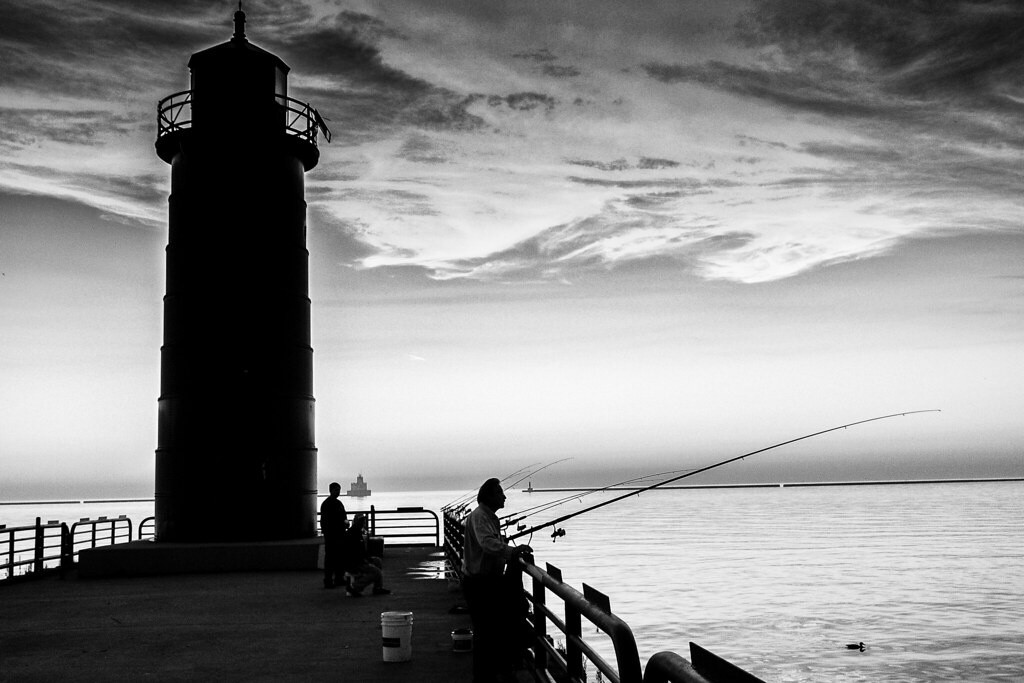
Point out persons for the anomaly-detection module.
[342,512,392,597]
[461,476,534,683]
[320,482,350,589]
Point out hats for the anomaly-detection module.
[477,478,500,503]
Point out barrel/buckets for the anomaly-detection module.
[380,609,414,662]
[450,629,473,652]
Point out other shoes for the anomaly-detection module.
[346,585,361,597]
[372,587,391,594]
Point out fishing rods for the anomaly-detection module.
[458,456,575,522]
[440,462,541,516]
[498,467,698,528]
[506,408,942,540]
